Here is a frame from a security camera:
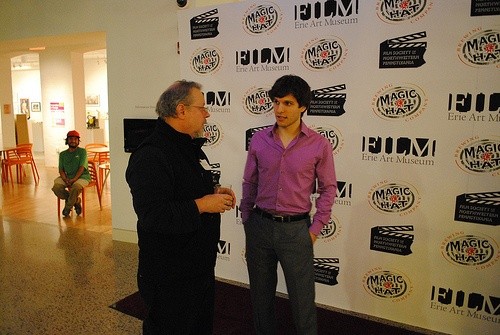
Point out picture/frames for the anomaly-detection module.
[20,98,30,119]
[85,95,100,106]
[31,102,41,112]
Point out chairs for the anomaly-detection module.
[58,161,103,221]
[85,144,110,166]
[0,144,40,188]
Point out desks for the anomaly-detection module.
[44,129,104,167]
[89,147,110,191]
[98,164,110,194]
[16,114,29,145]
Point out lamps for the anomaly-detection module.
[19,63,22,68]
[97,60,100,64]
[12,64,15,68]
[103,59,107,64]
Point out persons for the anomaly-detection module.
[125,79,236,335]
[239,74,338,335]
[51,130,91,217]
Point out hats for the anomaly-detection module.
[67,130,80,137]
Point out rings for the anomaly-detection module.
[224,200,227,205]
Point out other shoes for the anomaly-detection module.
[74,197,81,215]
[184,105,208,115]
[62,207,72,217]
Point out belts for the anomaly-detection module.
[256,205,309,222]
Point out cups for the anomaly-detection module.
[105,161,109,167]
[215,183,231,196]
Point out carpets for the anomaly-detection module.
[109,280,426,335]
